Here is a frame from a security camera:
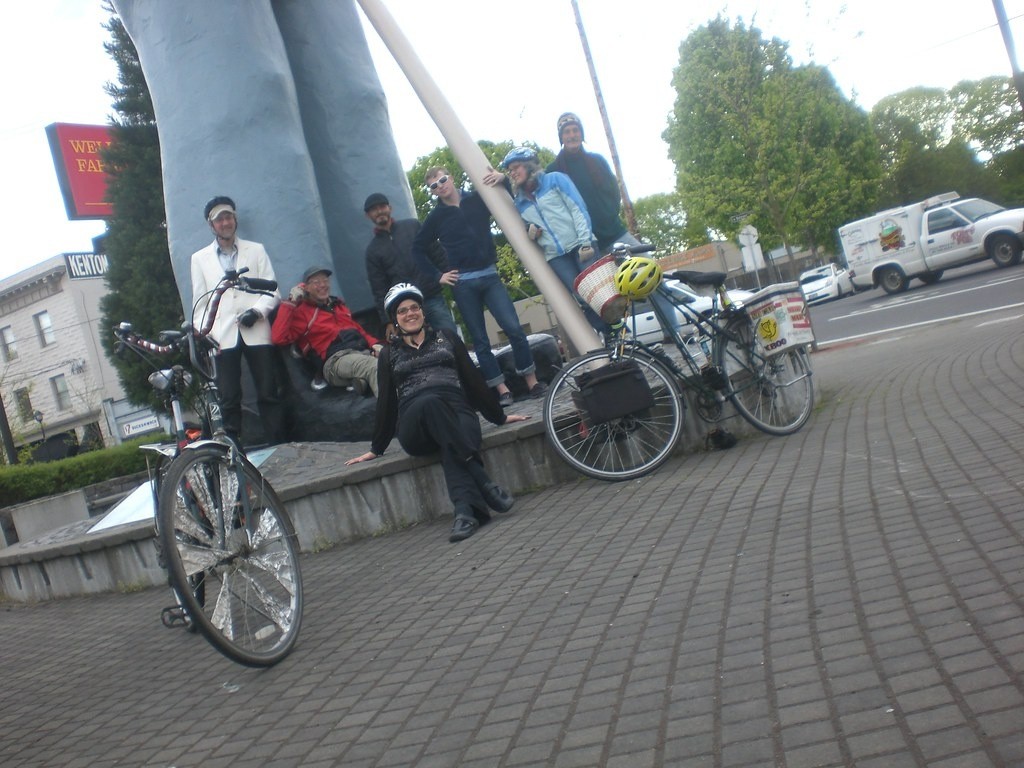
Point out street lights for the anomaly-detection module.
[32,410,46,442]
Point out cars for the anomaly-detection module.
[799,262,857,307]
[594,277,756,348]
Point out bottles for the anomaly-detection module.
[310,372,328,393]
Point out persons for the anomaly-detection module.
[412,167,550,407]
[364,193,449,342]
[501,113,695,361]
[271,265,383,398]
[345,283,531,542]
[191,196,282,452]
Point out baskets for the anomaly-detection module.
[573,253,627,324]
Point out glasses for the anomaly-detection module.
[430,175,449,190]
[307,277,330,287]
[396,303,421,315]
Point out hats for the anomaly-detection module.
[303,265,333,283]
[557,112,585,147]
[364,193,389,211]
[208,204,235,221]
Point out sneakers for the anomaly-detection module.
[449,520,476,542]
[482,482,514,514]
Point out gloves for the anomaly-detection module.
[289,286,304,304]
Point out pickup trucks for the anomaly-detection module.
[836,191,1024,296]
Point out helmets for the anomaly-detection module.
[203,196,236,220]
[501,147,537,169]
[385,282,423,315]
[614,257,663,301]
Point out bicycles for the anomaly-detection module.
[542,242,815,483]
[109,267,303,669]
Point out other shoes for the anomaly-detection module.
[499,392,513,406]
[529,382,547,398]
[352,379,368,397]
